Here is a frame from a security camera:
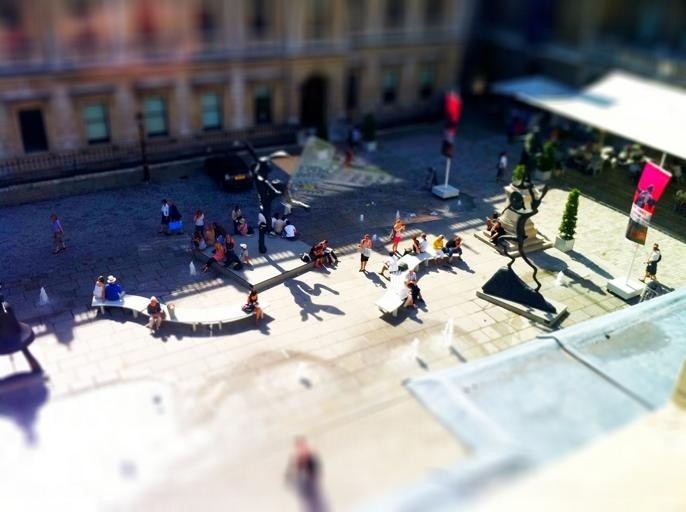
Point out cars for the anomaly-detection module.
[203,151,254,191]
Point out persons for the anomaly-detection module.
[486,212,505,246]
[288,436,333,512]
[359,233,370,274]
[49,214,66,253]
[92,274,125,305]
[378,218,463,309]
[496,147,509,183]
[147,295,162,332]
[248,289,264,325]
[638,243,661,283]
[310,239,339,269]
[157,198,298,273]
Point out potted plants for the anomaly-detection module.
[553,189,581,254]
[361,113,378,153]
[536,140,553,181]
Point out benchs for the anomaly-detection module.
[91,292,170,321]
[373,247,459,317]
